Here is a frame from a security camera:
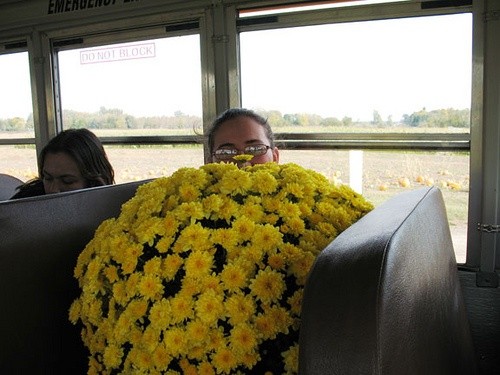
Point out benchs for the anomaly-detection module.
[297,185,500,375]
[0,172,25,202]
[0,177,154,375]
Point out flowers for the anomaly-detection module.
[66,153,374,375]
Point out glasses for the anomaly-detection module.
[211,143,274,161]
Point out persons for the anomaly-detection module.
[206,106,282,172]
[9,127,118,200]
